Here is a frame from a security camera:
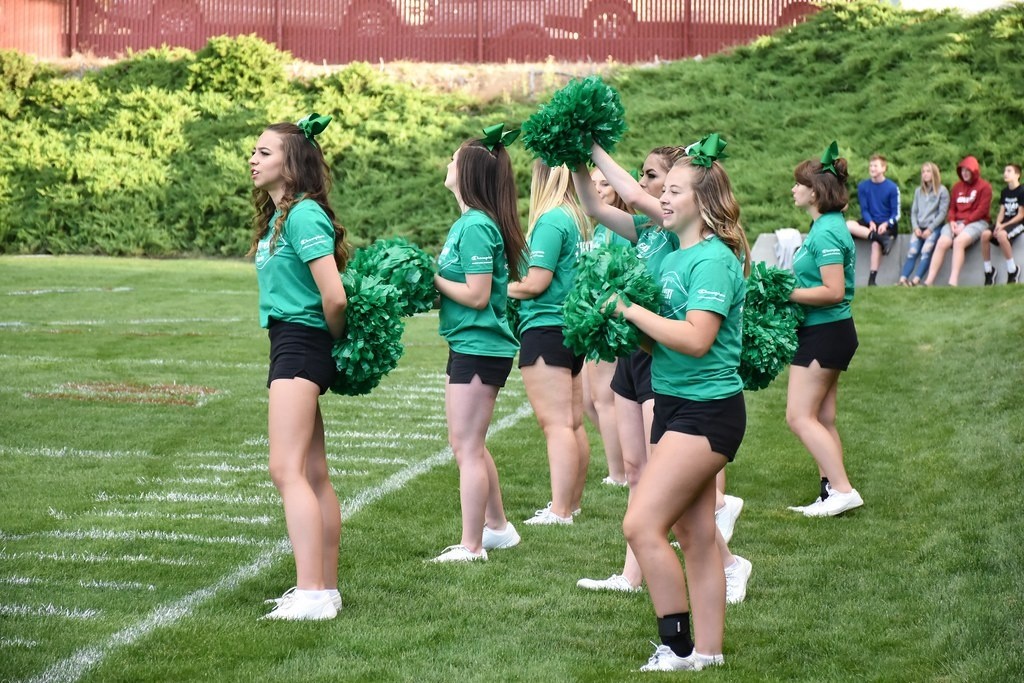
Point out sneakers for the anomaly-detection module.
[806,482,864,517]
[256,591,337,620]
[481,521,521,551]
[1007,265,1020,284]
[601,476,628,488]
[715,495,743,544]
[264,586,342,611]
[724,555,752,604]
[630,640,724,673]
[577,574,642,593]
[786,496,822,512]
[984,267,997,287]
[671,542,681,548]
[547,502,582,515]
[523,509,573,525]
[422,545,488,564]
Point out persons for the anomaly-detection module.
[981,164,1024,286]
[424,138,521,564]
[785,156,865,517]
[898,162,951,286]
[923,155,992,286]
[505,156,591,526]
[846,156,902,287]
[246,122,347,621]
[567,123,755,672]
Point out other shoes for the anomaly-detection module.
[880,236,894,255]
[895,280,914,288]
